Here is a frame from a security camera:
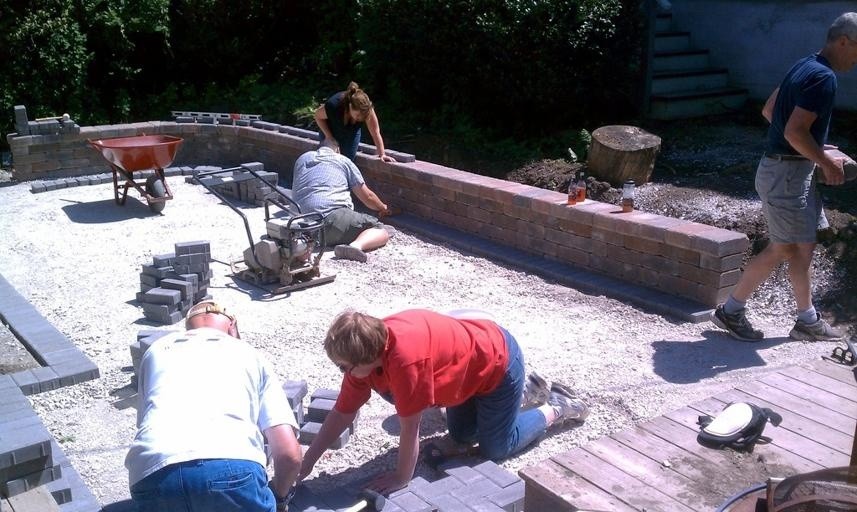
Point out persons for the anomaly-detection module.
[709,12,855,342]
[123,297,303,512]
[293,309,593,494]
[311,81,395,186]
[289,136,391,262]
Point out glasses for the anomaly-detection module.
[338,363,356,376]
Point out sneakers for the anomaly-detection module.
[524,370,552,405]
[709,302,764,342]
[788,313,846,341]
[334,244,368,263]
[547,380,590,425]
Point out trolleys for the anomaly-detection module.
[86,132,183,212]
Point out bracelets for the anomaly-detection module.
[377,152,386,157]
[268,479,297,506]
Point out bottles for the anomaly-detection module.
[576,170,585,202]
[622,177,635,213]
[567,174,576,205]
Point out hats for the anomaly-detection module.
[185,300,241,339]
